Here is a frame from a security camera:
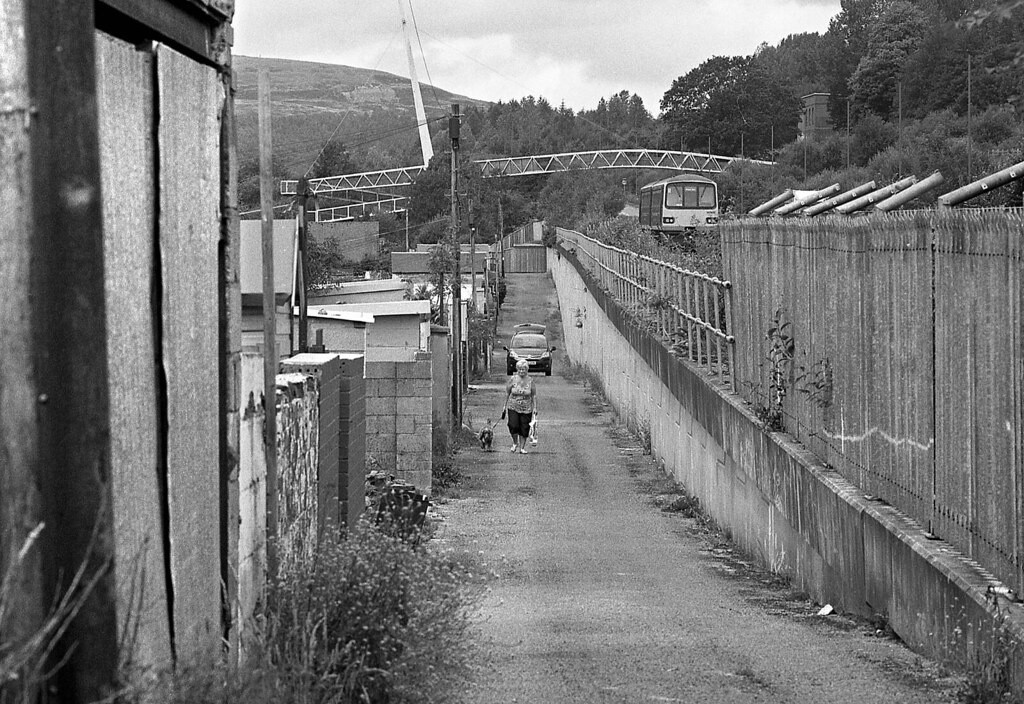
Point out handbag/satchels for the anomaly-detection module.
[529,414,538,446]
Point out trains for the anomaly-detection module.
[638,173,719,236]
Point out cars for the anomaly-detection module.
[502,323,556,376]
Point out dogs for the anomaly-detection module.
[478,417,494,452]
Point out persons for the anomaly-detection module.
[501,360,538,455]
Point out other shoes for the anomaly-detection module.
[511,444,518,452]
[520,449,527,454]
